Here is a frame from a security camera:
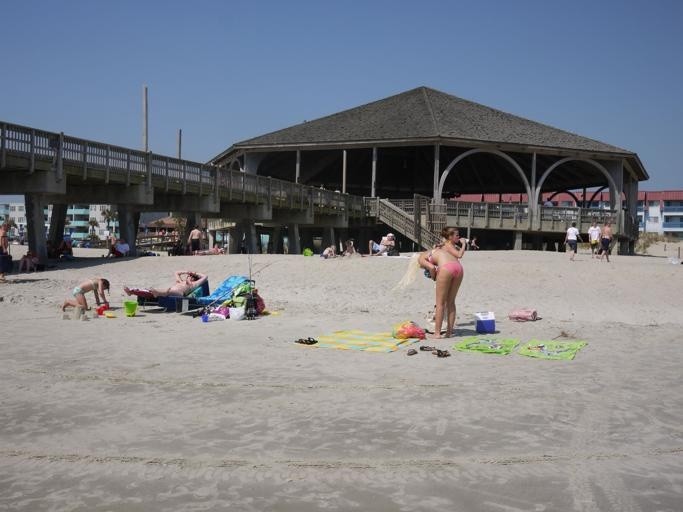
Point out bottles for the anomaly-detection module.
[457,238,469,245]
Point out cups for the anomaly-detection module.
[201,314,208,323]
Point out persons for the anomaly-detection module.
[598,221,614,262]
[418,249,463,339]
[587,220,602,259]
[460,237,481,250]
[187,224,225,256]
[101,234,131,259]
[434,226,467,322]
[18,251,38,273]
[122,270,208,299]
[563,222,583,260]
[544,197,553,207]
[322,232,396,261]
[61,279,109,314]
[0,216,17,251]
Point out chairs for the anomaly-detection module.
[136,277,211,311]
[192,274,256,320]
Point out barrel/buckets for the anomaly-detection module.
[123,301,138,317]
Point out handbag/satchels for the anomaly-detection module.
[425,311,448,333]
[387,249,400,256]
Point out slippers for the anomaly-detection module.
[432,350,450,357]
[408,349,417,355]
[420,345,432,351]
[295,338,317,344]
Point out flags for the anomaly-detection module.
[643,192,651,221]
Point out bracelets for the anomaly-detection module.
[181,271,184,274]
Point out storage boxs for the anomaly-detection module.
[473,311,497,334]
